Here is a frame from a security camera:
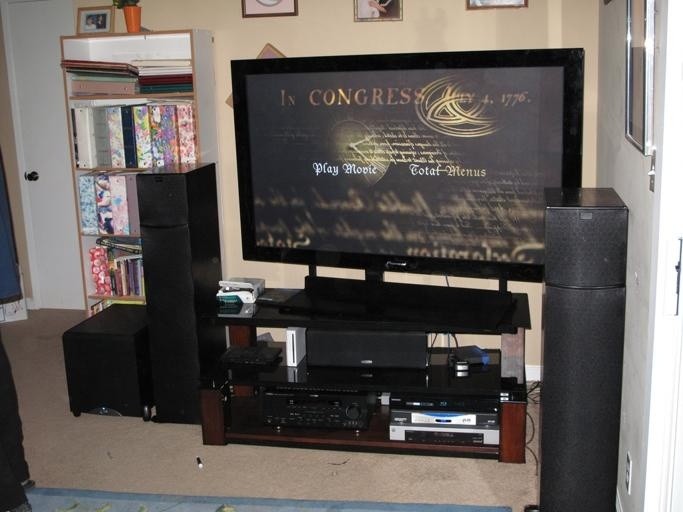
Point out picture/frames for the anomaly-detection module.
[241,0,299,18]
[354,0,404,22]
[624,0,654,155]
[467,0,529,11]
[76,5,116,35]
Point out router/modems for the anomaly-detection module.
[454,345,490,365]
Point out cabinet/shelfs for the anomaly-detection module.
[60,27,219,321]
[198,285,531,464]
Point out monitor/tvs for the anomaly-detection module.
[232,47,586,329]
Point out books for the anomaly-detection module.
[69,99,199,170]
[78,170,152,237]
[59,60,193,96]
[89,236,146,316]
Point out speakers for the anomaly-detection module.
[136,164,226,426]
[62,303,156,421]
[543,187,628,511]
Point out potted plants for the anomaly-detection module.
[113,0,141,33]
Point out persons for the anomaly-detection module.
[83,15,106,29]
